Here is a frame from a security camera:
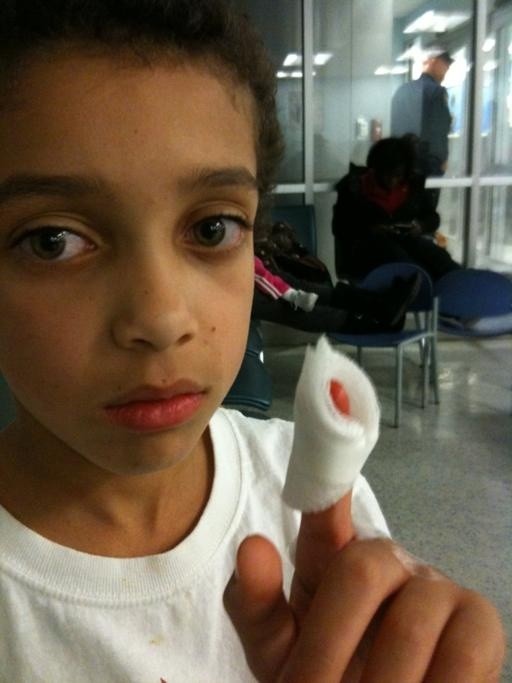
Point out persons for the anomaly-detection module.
[0,0,508,683]
[388,49,455,208]
[329,132,460,284]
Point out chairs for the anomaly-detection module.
[254,187,512,430]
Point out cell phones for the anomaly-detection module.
[394,224,412,234]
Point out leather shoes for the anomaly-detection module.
[379,271,421,334]
